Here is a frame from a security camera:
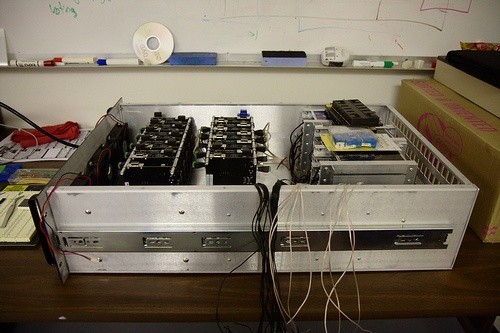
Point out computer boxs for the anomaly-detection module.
[32,95,478,282]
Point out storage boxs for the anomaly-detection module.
[398,77,500,243]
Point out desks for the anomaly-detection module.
[0,127,500,333]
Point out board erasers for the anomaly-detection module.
[261,50,308,67]
[168,52,218,65]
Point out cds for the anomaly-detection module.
[132,22,174,64]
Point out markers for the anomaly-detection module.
[9,60,55,67]
[97,58,140,67]
[351,60,398,68]
[52,57,96,66]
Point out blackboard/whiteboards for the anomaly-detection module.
[0,0,500,70]
